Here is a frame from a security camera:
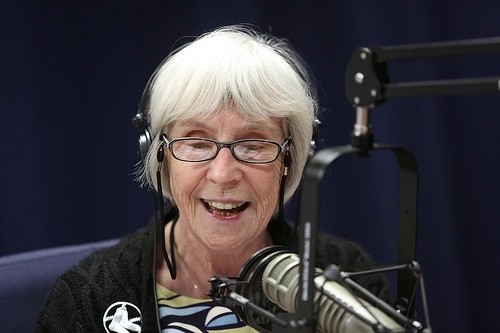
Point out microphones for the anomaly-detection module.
[262,253,405,333]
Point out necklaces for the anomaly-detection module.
[190,276,205,299]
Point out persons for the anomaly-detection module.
[33,22,392,332]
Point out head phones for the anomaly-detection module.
[131,42,320,174]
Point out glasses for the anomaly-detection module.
[157,131,293,164]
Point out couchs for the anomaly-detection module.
[0,238,120,333]
